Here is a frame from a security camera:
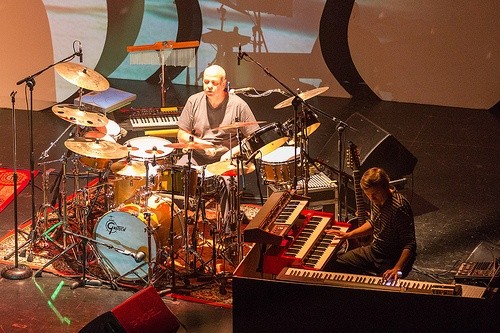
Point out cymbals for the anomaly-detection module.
[54,61,109,91]
[64,136,128,159]
[52,104,108,127]
[273,86,330,109]
[206,159,255,176]
[111,160,148,176]
[209,121,268,132]
[163,142,222,149]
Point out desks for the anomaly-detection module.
[266,171,338,221]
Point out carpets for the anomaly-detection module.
[0,176,263,309]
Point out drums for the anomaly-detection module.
[83,115,120,143]
[154,164,198,201]
[114,158,157,210]
[123,136,174,194]
[241,121,289,159]
[91,192,184,281]
[284,109,322,146]
[257,147,306,184]
[79,130,116,172]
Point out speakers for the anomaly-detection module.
[318,113,417,190]
[77,284,180,333]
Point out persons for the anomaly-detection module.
[324,168,417,281]
[167,65,260,234]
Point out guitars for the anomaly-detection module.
[344,139,375,253]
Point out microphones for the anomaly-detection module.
[79,42,83,63]
[245,150,260,165]
[238,43,241,65]
[134,251,146,264]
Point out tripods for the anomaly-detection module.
[3,89,251,297]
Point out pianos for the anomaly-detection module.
[113,105,184,130]
[243,190,448,296]
